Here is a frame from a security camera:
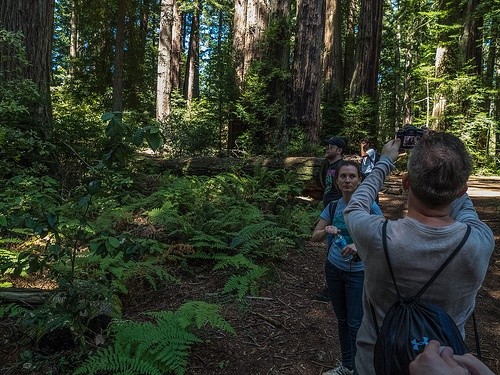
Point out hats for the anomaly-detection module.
[322,137,346,152]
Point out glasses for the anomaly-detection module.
[329,144,339,148]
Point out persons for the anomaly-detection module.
[312,161,385,375]
[321,136,346,249]
[359,136,378,205]
[410,340,495,375]
[343,126,494,375]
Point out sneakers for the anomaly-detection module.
[322,359,354,375]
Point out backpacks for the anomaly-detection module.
[369,219,481,375]
[367,148,380,166]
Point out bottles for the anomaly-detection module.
[334,234,353,261]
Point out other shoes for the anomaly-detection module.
[316,288,332,301]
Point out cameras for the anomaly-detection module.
[395,124,424,150]
